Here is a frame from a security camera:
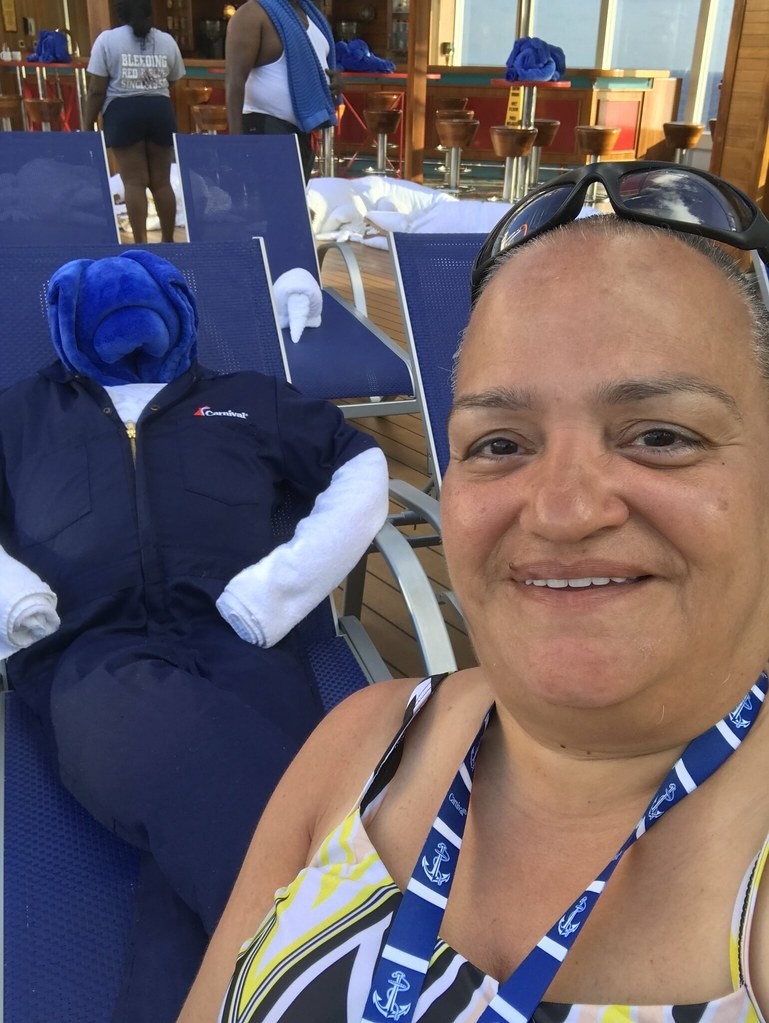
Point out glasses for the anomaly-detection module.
[473,162,769,306]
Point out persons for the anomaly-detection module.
[175,159,769,1023]
[83,0,186,245]
[225,0,343,186]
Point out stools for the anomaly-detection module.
[487,118,560,203]
[24,100,62,131]
[663,122,705,164]
[433,97,481,194]
[0,94,23,131]
[317,105,346,177]
[574,125,621,204]
[182,86,229,135]
[364,94,403,174]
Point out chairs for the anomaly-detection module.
[0,127,119,248]
[1,233,458,1023]
[172,133,424,417]
[388,229,491,550]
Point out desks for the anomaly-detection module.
[490,78,571,200]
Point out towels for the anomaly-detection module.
[25,28,72,62]
[271,267,324,344]
[333,38,396,72]
[0,540,60,663]
[45,250,201,382]
[215,445,388,647]
[256,1,345,132]
[504,36,567,83]
